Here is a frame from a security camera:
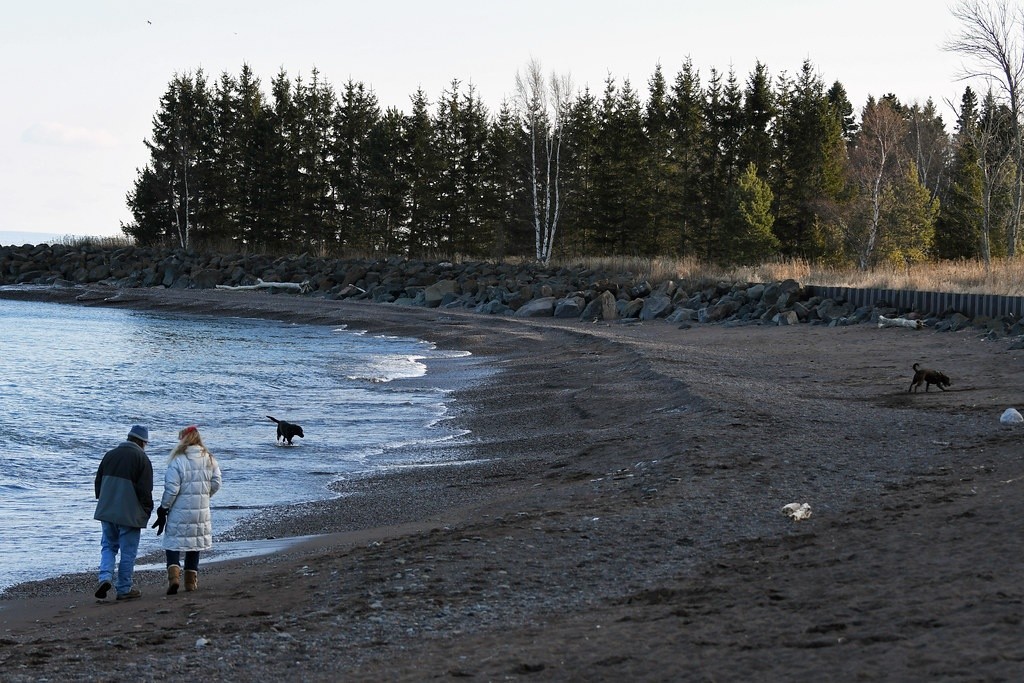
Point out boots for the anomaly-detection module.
[183,569,198,590]
[166,564,181,595]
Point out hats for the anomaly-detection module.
[128,424,149,442]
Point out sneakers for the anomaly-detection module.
[94,580,112,598]
[116,588,142,600]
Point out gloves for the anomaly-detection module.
[152,507,168,536]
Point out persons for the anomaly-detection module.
[94,426,155,601]
[152,426,222,594]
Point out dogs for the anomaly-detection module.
[266,416,304,445]
[908,363,951,392]
